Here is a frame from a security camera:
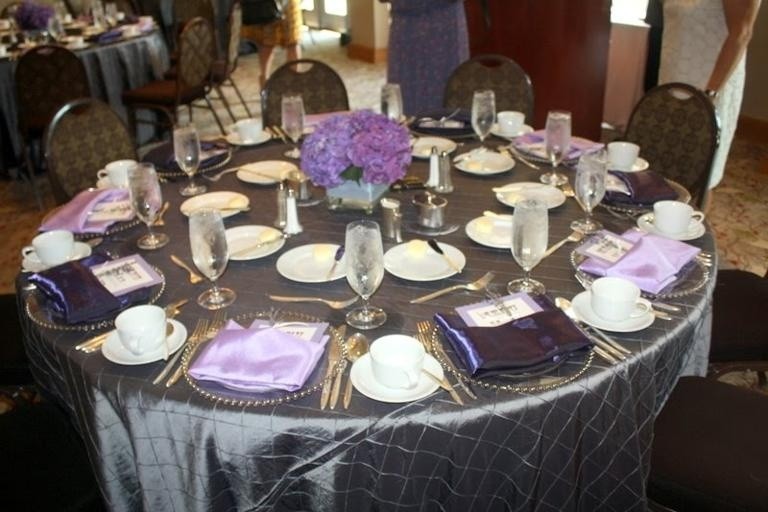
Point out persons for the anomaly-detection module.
[237,1,303,91]
[657,1,761,220]
[387,0,470,116]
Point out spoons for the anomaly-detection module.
[555,296,632,354]
[344,333,368,409]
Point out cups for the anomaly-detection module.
[381,83,402,122]
[173,122,207,196]
[115,305,167,355]
[539,110,572,186]
[189,207,237,309]
[281,93,306,159]
[369,334,425,390]
[497,111,525,134]
[591,277,651,321]
[344,220,387,331]
[653,200,705,233]
[97,159,138,188]
[506,200,548,297]
[128,163,169,250]
[21,230,73,266]
[607,141,645,169]
[570,148,608,234]
[471,89,496,151]
[229,118,262,141]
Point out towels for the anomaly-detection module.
[100,31,121,42]
[188,319,329,393]
[29,253,153,324]
[305,111,354,128]
[38,187,135,232]
[435,293,595,380]
[516,128,605,161]
[578,228,702,296]
[414,104,480,133]
[603,166,679,204]
[144,140,223,172]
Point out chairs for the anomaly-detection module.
[11,44,90,211]
[622,81,722,210]
[645,375,768,512]
[708,268,768,378]
[260,59,350,131]
[443,53,534,130]
[42,97,140,203]
[1,397,109,511]
[121,16,226,143]
[168,0,253,123]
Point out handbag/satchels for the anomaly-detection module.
[241,1,285,25]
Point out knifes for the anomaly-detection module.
[320,323,347,409]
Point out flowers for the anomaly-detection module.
[299,108,413,187]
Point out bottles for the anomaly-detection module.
[434,150,454,193]
[283,190,304,234]
[273,182,287,229]
[426,146,439,187]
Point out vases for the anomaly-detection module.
[324,180,389,215]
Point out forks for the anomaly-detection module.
[269,295,360,309]
[510,148,540,170]
[166,310,227,387]
[410,271,495,304]
[562,184,593,217]
[544,228,584,258]
[575,271,680,311]
[417,320,478,400]
[154,201,170,226]
[583,281,673,321]
[414,334,464,406]
[170,255,206,284]
[153,318,209,384]
[202,166,242,182]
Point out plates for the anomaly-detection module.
[490,124,534,141]
[237,160,298,186]
[453,152,516,177]
[383,242,466,281]
[572,291,655,331]
[276,243,346,283]
[182,311,347,406]
[156,150,232,177]
[465,214,513,248]
[512,136,594,164]
[48,201,141,240]
[409,136,457,160]
[496,182,566,209]
[570,248,708,299]
[408,127,478,139]
[22,241,92,272]
[101,318,188,365]
[180,190,250,219]
[350,351,444,403]
[225,225,286,260]
[26,264,165,331]
[598,177,691,213]
[226,131,271,146]
[637,212,706,240]
[432,324,595,392]
[591,152,649,173]
